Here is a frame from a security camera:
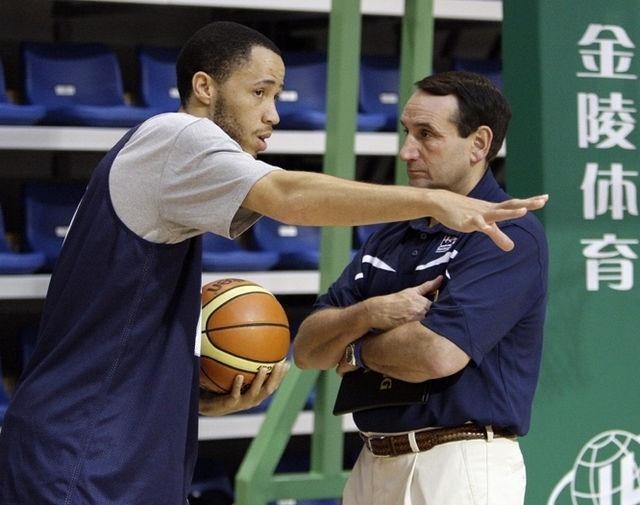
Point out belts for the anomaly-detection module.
[358,418,515,459]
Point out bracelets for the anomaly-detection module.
[345,339,366,371]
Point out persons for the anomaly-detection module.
[2,18,553,505]
[290,67,549,505]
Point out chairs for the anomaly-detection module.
[1,299,366,504]
[1,149,395,273]
[1,46,506,131]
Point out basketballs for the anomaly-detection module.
[198,279,291,393]
